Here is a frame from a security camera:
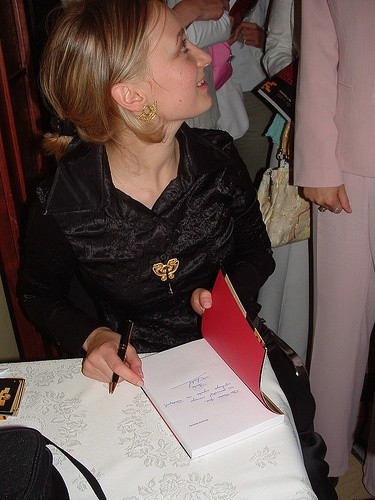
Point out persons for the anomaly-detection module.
[40,0,340,500]
[293,0,374,495]
[166,0,313,371]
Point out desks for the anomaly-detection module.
[0,346,318,500]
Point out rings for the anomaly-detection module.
[318,206,326,212]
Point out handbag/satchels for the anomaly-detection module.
[0,426,105,500]
[256,120,309,248]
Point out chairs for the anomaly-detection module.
[0,0,311,385]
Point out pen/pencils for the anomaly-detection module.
[110,320,134,393]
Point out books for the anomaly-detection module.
[132,270,285,461]
